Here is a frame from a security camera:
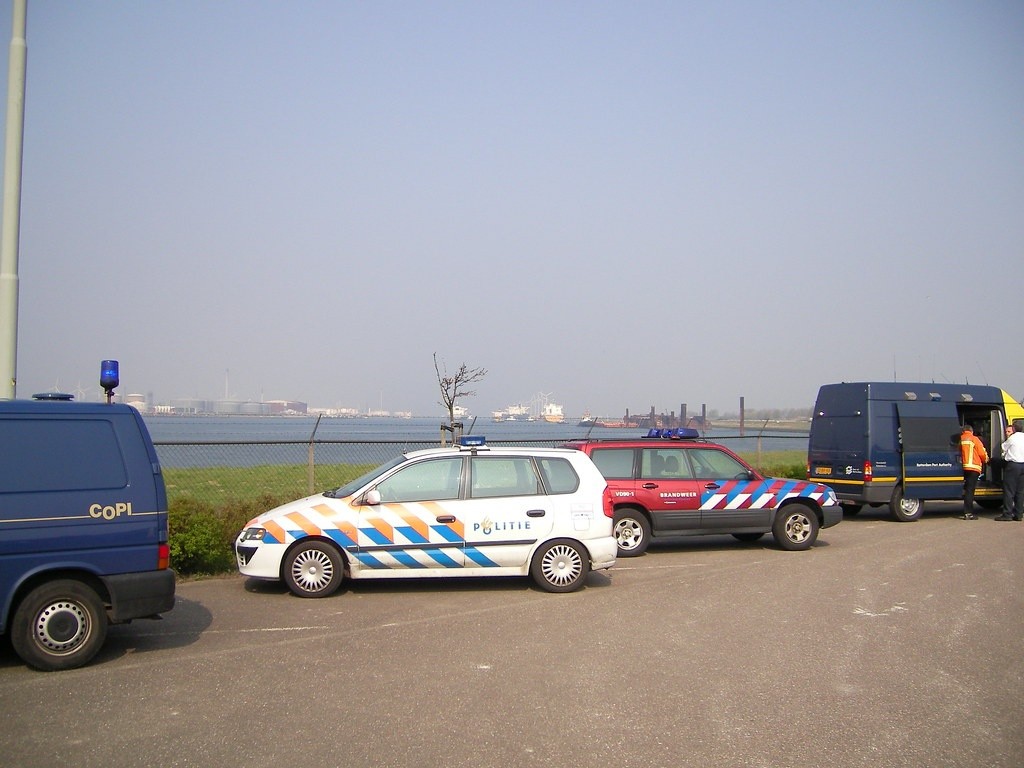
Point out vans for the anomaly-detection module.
[807,383,1024,522]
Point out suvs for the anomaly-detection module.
[561,429,848,559]
[0,358,178,672]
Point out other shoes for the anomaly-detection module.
[995,514,1013,521]
[1013,515,1022,521]
[965,513,978,520]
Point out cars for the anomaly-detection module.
[235,433,619,600]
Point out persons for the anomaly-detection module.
[994,421,1024,521]
[960,425,990,520]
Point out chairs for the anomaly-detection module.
[658,455,679,475]
[975,422,991,450]
[449,460,475,490]
